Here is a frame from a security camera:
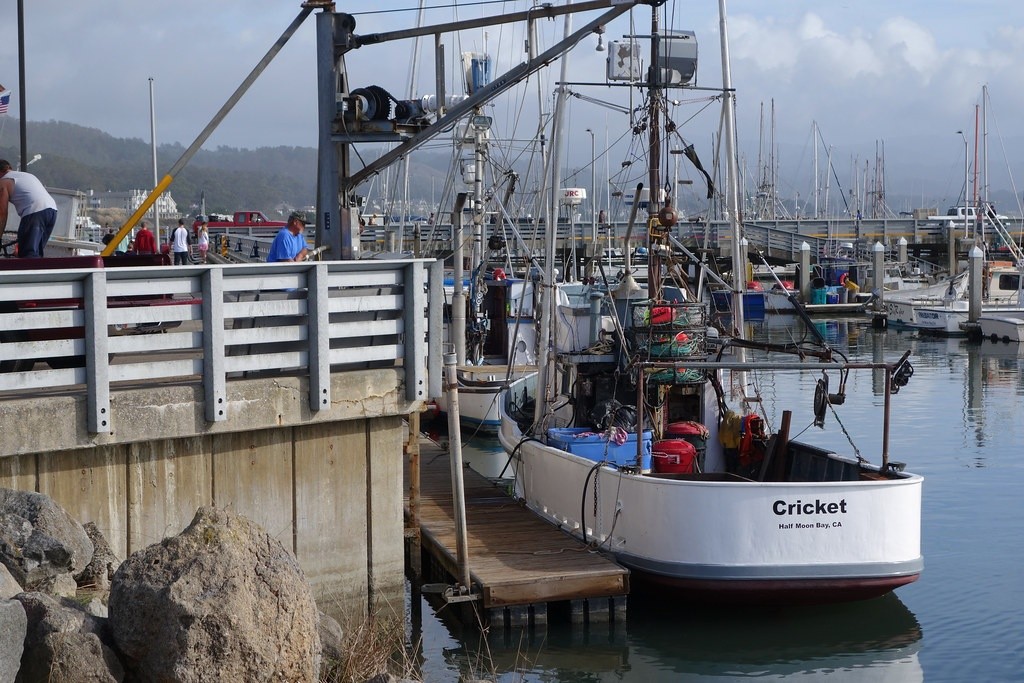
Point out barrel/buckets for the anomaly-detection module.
[655,421,709,474]
[836,287,858,303]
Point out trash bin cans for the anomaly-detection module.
[810,277,827,304]
[652,439,696,474]
[667,421,709,472]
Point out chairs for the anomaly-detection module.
[188,253,205,265]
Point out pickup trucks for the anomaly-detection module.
[926,204,1011,235]
[193,211,288,238]
[0,253,175,375]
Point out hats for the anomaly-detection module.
[431,212,434,215]
[288,210,312,224]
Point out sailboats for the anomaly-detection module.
[496,0,926,614]
[343,0,1024,442]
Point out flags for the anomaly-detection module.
[0,95,9,113]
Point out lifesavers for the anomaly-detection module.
[492,268,507,282]
[840,273,850,287]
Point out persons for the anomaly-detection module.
[134,222,157,255]
[102,229,114,256]
[266,212,311,291]
[0,159,58,258]
[169,219,193,265]
[198,222,209,263]
[115,226,128,256]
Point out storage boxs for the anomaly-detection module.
[811,287,827,304]
[827,292,839,303]
[548,427,653,474]
[856,293,873,303]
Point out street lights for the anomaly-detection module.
[957,130,968,239]
[148,77,161,253]
[586,128,596,259]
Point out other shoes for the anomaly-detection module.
[204,260,207,264]
